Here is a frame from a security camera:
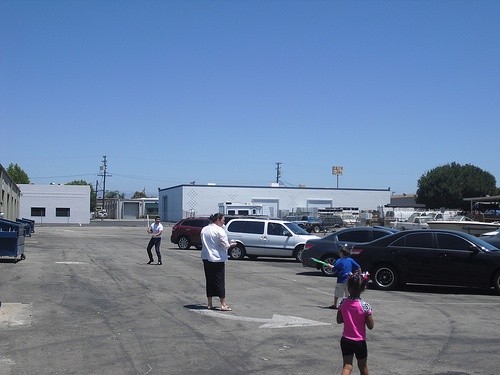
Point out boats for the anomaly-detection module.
[389,209,500,237]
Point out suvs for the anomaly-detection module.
[224,218,322,263]
[170,216,212,250]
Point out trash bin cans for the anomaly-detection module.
[22,217,35,232]
[16,217,32,237]
[0,218,26,262]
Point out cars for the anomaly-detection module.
[477,229,500,249]
[285,212,344,233]
[349,228,500,296]
[302,225,401,277]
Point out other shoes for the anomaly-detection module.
[147,258,154,264]
[158,261,162,265]
[221,306,232,311]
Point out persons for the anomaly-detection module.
[329,249,361,309]
[201,213,237,311]
[337,274,374,375]
[146,216,163,265]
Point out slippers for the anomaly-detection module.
[329,305,338,309]
